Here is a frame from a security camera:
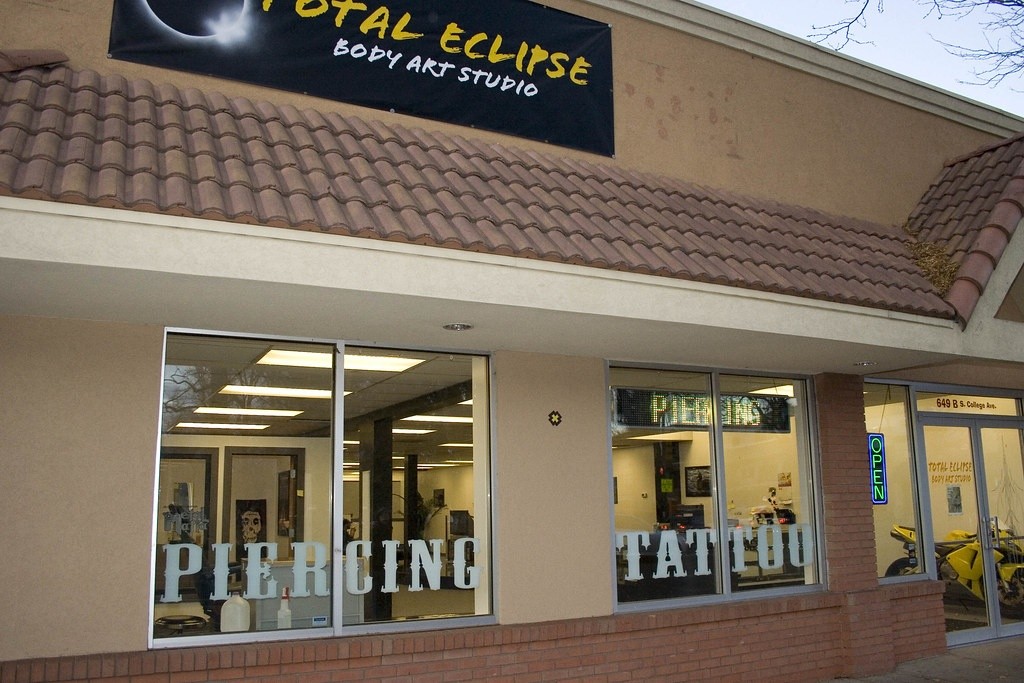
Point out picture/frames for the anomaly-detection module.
[278,470,290,536]
[433,489,444,508]
[684,466,712,497]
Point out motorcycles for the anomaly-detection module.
[884,514,1024,620]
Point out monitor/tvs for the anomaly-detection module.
[450,510,474,538]
[675,504,704,529]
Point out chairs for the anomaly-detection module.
[169,504,243,631]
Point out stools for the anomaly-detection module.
[154,614,206,637]
